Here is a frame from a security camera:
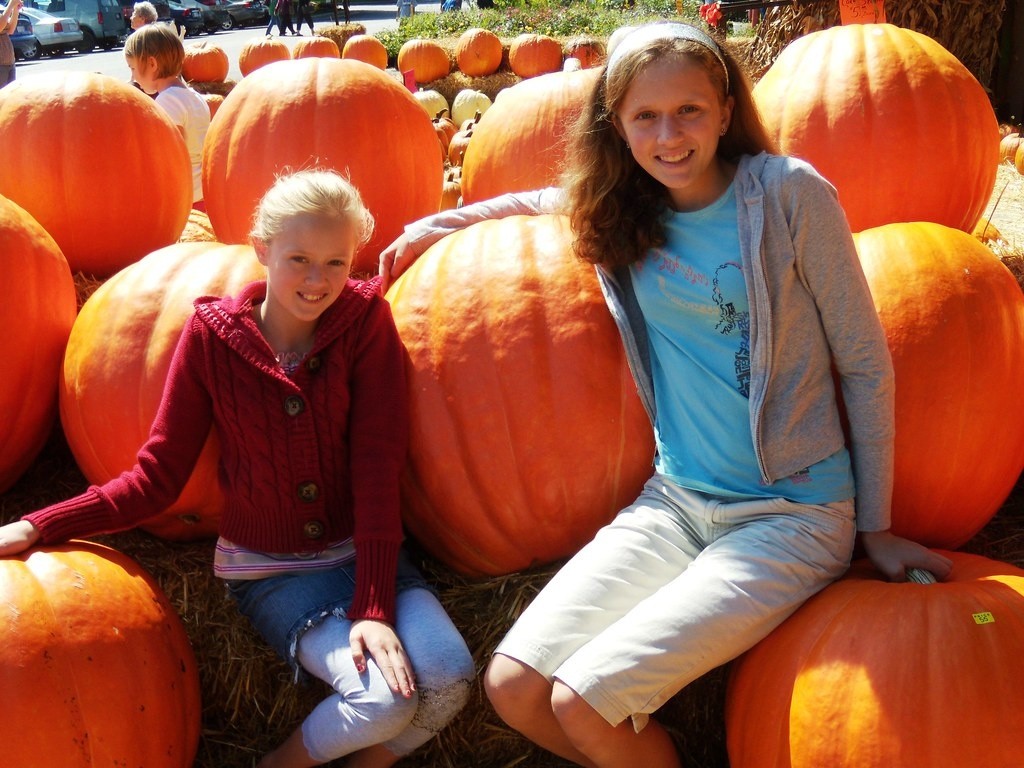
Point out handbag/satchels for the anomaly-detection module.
[263,0,270,8]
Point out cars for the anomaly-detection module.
[166,0,270,40]
[0,3,37,61]
[21,6,83,60]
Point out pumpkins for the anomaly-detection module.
[0,24,1024,768]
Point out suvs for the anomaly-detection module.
[7,0,169,54]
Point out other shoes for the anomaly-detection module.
[297,32,303,36]
[292,32,297,35]
[266,33,273,39]
[279,32,287,36]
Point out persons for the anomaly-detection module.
[0,172,476,768]
[396,0,417,20]
[483,28,954,766]
[123,26,210,213]
[130,2,159,100]
[442,0,459,11]
[0,0,25,89]
[265,0,315,36]
